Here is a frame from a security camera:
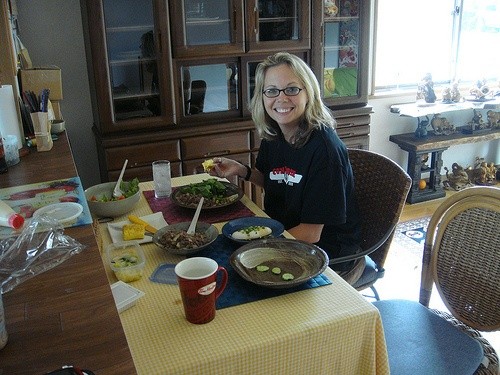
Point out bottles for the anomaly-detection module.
[0,199,24,230]
[325,0,339,17]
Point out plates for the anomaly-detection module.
[32,202,82,224]
[230,239,330,290]
[106,211,168,248]
[222,216,284,245]
[152,221,219,254]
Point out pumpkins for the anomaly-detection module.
[419,180,427,189]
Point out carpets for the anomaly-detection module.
[393,216,440,262]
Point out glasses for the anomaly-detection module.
[262,87,306,98]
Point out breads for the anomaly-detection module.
[123,224,146,240]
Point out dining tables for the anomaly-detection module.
[83,172,391,375]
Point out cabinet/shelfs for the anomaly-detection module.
[388,94,500,205]
[79,0,374,198]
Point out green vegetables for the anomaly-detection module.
[178,178,226,204]
[96,178,139,202]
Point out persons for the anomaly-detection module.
[138,31,160,115]
[208,52,357,271]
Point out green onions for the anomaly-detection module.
[238,225,265,237]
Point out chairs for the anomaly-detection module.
[370,186,500,375]
[266,148,412,300]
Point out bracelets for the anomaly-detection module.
[244,165,251,181]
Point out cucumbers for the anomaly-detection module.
[257,265,270,272]
[271,267,281,275]
[282,273,294,280]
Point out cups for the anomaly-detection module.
[174,257,228,325]
[152,160,172,199]
[49,119,65,133]
[3,135,19,168]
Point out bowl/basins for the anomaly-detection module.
[84,182,141,218]
[169,180,245,213]
[105,240,146,282]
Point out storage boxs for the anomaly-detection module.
[18,64,63,101]
[50,102,63,120]
